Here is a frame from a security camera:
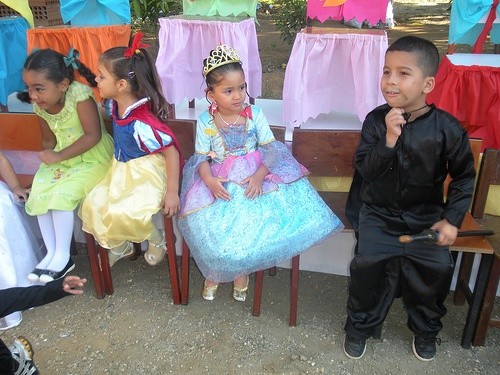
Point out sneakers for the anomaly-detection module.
[7,336,39,375]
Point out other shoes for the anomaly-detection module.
[143,229,177,266]
[201,277,218,301]
[343,333,368,360]
[40,256,75,282]
[412,334,441,361]
[5,311,23,327]
[232,275,250,302]
[96,241,133,271]
[27,267,44,282]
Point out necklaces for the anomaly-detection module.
[402,105,429,119]
[216,109,241,125]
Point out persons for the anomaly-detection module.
[78,31,184,271]
[177,43,345,303]
[0,151,89,375]
[343,36,477,361]
[21,47,115,285]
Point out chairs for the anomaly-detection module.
[288,126,369,329]
[27,0,131,103]
[177,125,287,317]
[1,112,105,300]
[443,136,494,349]
[284,0,393,127]
[426,0,500,149]
[449,148,500,348]
[155,0,262,108]
[97,120,196,306]
